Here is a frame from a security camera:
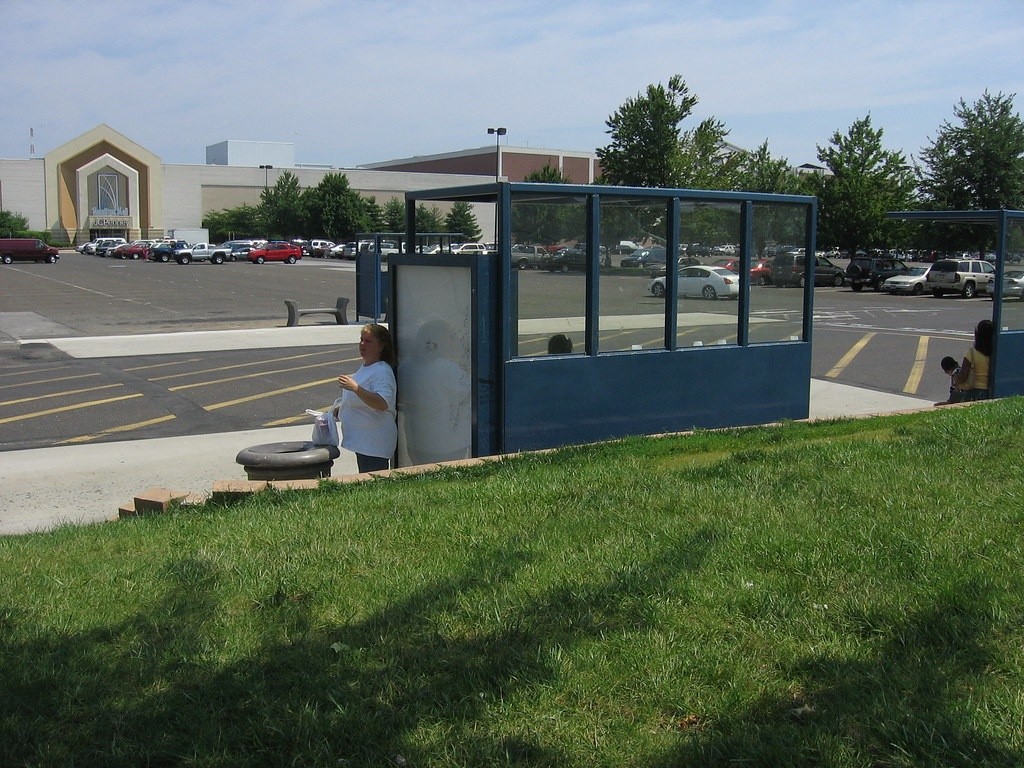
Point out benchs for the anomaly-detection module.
[284,297,350,327]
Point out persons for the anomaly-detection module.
[955,320,995,402]
[143,243,152,262]
[332,324,397,473]
[933,356,966,406]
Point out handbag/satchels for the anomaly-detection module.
[303,397,340,447]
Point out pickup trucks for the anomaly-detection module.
[510,244,549,267]
[0,236,60,264]
[173,243,232,265]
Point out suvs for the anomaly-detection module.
[770,251,844,288]
[843,258,908,292]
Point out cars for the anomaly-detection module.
[247,241,302,264]
[985,270,1024,303]
[927,258,997,299]
[450,243,487,255]
[148,242,177,262]
[883,264,932,296]
[649,265,740,299]
[231,247,252,261]
[343,241,371,259]
[545,248,602,273]
[619,247,666,267]
[714,257,740,273]
[750,257,772,286]
[646,256,704,278]
[73,233,345,258]
[412,220,1000,259]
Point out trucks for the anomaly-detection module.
[369,241,406,261]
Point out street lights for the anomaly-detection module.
[487,123,508,180]
[260,162,273,200]
